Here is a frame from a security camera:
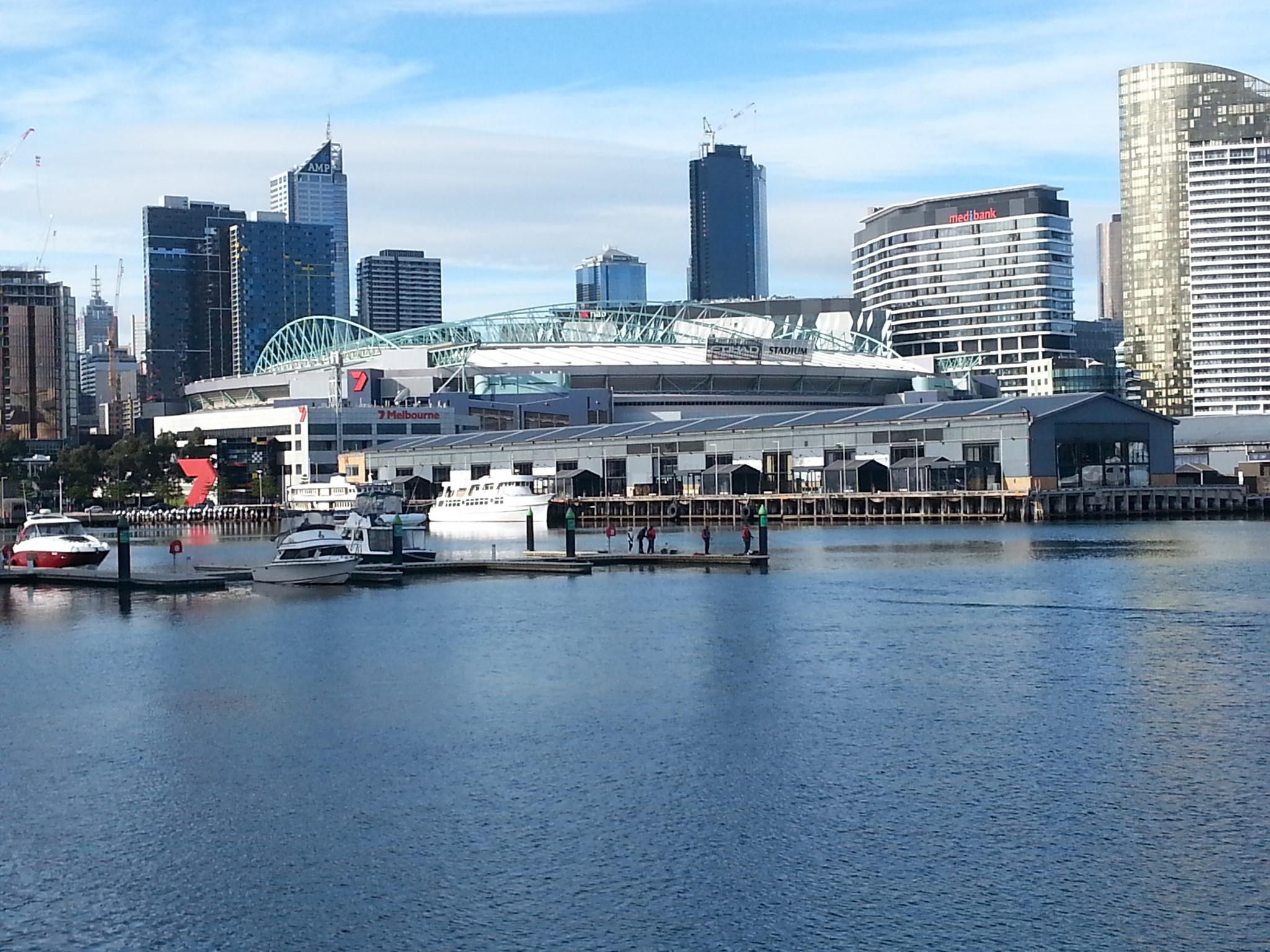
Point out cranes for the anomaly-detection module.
[0,127,41,169]
[103,258,125,402]
[37,214,57,269]
[703,101,757,153]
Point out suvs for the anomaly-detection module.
[85,505,103,514]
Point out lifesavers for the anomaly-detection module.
[668,503,681,519]
[741,506,753,521]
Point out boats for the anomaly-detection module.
[427,453,555,522]
[250,487,436,585]
[0,476,111,568]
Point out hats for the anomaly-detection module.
[645,527,647,529]
[744,525,748,529]
[628,527,633,531]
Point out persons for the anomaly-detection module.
[741,525,754,556]
[627,527,634,554]
[701,526,712,555]
[637,525,656,554]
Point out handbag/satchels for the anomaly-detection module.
[630,539,634,544]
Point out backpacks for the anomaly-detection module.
[742,530,747,538]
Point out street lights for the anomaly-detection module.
[773,440,779,495]
[836,442,846,490]
[653,446,660,497]
[992,430,1003,490]
[909,438,919,491]
[1,476,7,518]
[311,461,318,482]
[709,443,718,495]
